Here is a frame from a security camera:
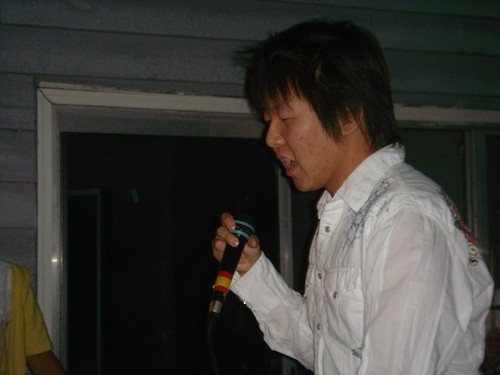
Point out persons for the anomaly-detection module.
[211,18,494,375]
[1,260,69,375]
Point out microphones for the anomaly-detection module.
[207,210,255,323]
